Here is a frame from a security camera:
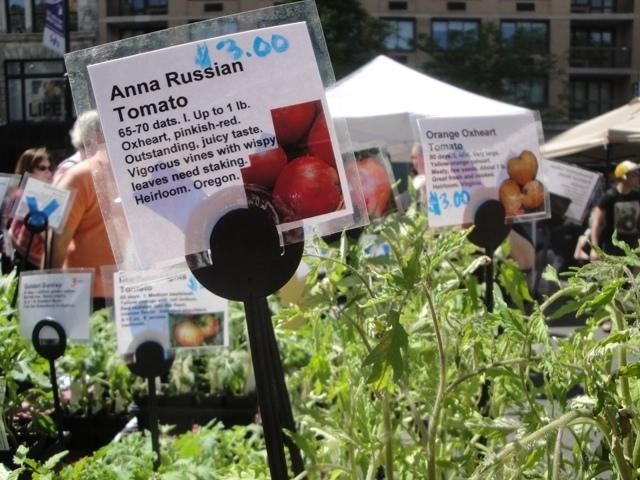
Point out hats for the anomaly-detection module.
[615,160,640,178]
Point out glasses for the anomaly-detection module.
[36,166,53,171]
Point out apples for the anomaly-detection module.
[345,157,390,215]
[499,152,543,214]
[241,100,344,223]
[174,314,219,346]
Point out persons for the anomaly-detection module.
[494,160,640,333]
[52,111,106,184]
[0,148,52,276]
[409,141,426,204]
[41,107,130,312]
